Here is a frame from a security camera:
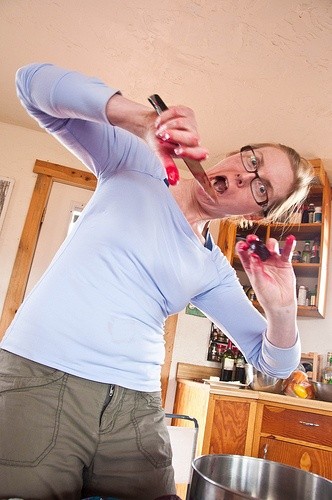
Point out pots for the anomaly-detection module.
[310,381,332,401]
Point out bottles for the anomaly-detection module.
[302,240,310,263]
[325,358,332,384]
[297,286,307,306]
[220,339,235,381]
[314,207,322,223]
[321,352,332,383]
[235,350,245,383]
[310,236,320,263]
[302,206,309,223]
[308,203,315,222]
[309,284,317,306]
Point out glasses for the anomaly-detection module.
[240,145,269,218]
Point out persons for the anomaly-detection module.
[0,64,315,500]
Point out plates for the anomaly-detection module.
[202,378,248,389]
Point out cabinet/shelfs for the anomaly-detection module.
[217,157,332,319]
[171,378,332,500]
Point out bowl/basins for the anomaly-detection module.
[189,454,332,500]
[248,367,283,394]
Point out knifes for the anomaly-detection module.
[148,94,220,208]
